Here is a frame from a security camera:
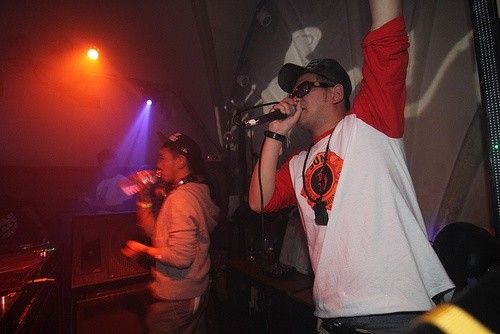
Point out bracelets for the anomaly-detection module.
[135,202,152,208]
[264,130,285,142]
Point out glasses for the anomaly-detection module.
[289,80,336,98]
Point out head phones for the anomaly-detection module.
[158,172,195,192]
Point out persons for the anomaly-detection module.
[247,0,457,334]
[0,133,220,334]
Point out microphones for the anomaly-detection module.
[234,104,297,128]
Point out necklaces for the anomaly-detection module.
[302,131,334,226]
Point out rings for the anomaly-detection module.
[273,105,276,111]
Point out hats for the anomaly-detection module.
[156,129,201,163]
[278,59,352,97]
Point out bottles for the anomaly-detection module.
[119,170,161,196]
